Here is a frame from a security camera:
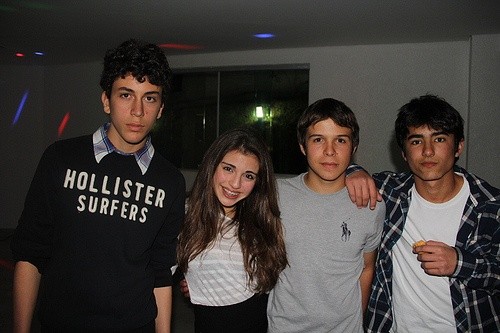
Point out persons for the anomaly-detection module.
[176,128,287,333]
[344,94,500,333]
[12,39,186,333]
[180,97,386,333]
